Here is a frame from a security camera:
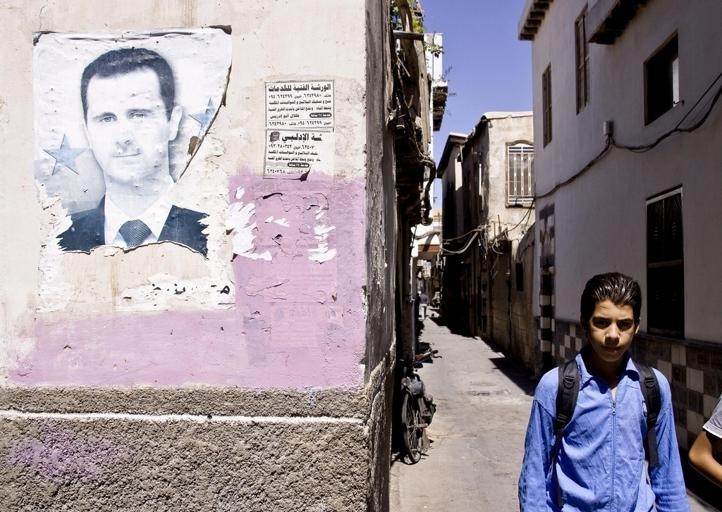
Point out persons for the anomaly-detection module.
[55,44,212,259]
[688,388,721,485]
[514,270,693,512]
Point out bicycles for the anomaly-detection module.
[393,345,444,464]
